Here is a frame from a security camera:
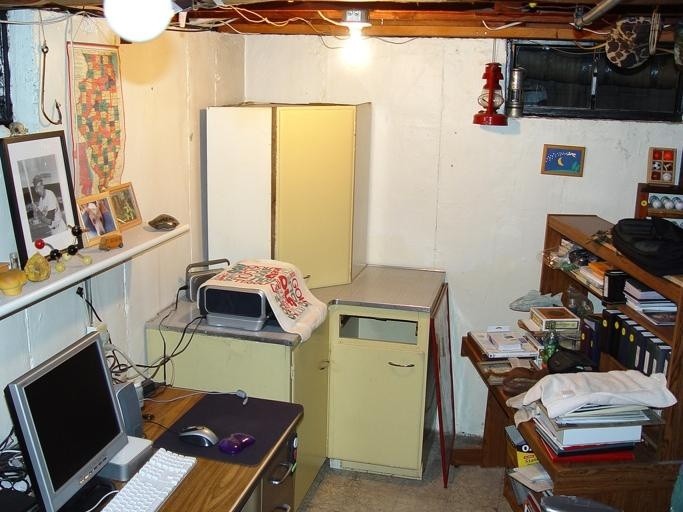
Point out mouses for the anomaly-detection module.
[176,424,219,449]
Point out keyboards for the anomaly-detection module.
[94,445,197,512]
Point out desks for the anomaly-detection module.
[460,336,683,512]
[64,384,303,511]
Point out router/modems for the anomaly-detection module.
[96,435,154,482]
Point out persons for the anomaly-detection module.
[28,199,34,218]
[32,174,67,236]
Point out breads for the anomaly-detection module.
[0,269,28,297]
[24,251,51,282]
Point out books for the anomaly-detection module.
[508,465,553,512]
[467,305,582,360]
[532,400,666,464]
[549,236,678,327]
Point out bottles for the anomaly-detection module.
[543,321,559,364]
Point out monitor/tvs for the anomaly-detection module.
[2,330,129,512]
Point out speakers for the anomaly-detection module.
[111,378,144,437]
[185,259,232,303]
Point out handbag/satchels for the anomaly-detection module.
[612,217,682,278]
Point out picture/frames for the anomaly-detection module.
[76,192,122,248]
[431,283,456,488]
[0,131,83,269]
[647,146,677,184]
[124,228,201,373]
[107,182,142,231]
[541,144,585,177]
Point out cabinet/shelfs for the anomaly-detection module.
[144,316,329,512]
[86,259,132,366]
[1,282,88,446]
[204,101,372,288]
[634,183,683,219]
[325,303,437,480]
[539,213,683,396]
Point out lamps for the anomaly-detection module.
[474,38,507,126]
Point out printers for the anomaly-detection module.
[198,285,274,332]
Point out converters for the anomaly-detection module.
[141,379,155,393]
